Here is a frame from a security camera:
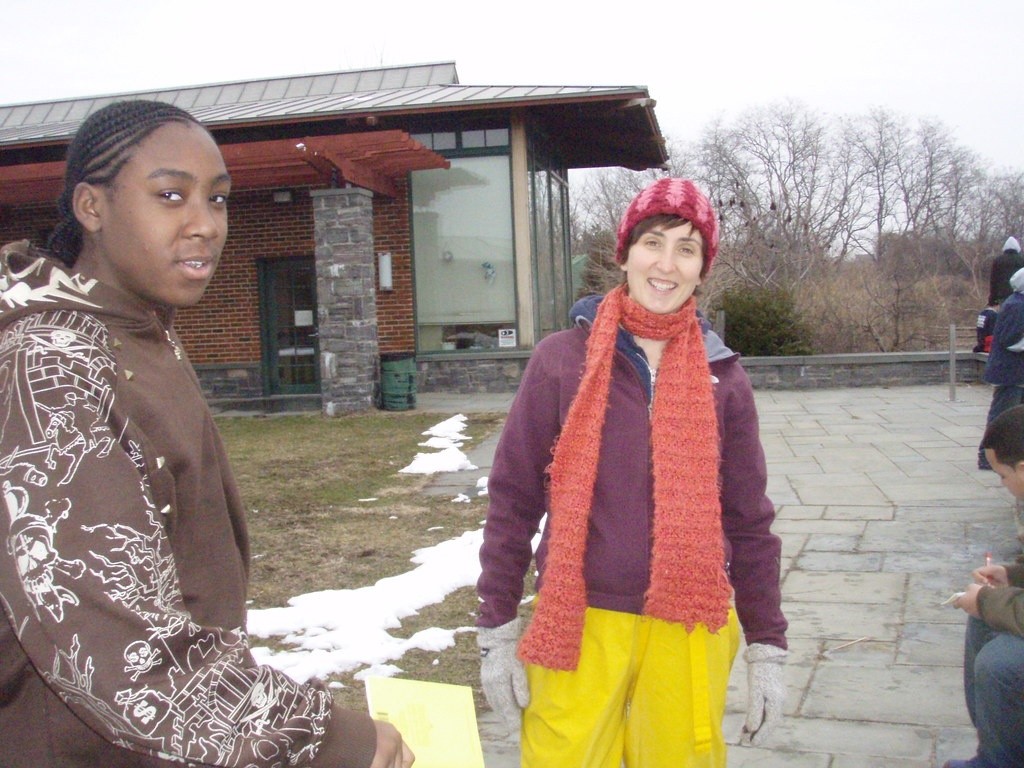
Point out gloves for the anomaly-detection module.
[736,643,790,745]
[476,616,532,730]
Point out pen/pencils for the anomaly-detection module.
[984,552,993,588]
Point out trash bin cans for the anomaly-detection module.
[379,351,417,411]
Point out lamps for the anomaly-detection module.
[378,250,393,291]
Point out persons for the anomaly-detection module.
[472,178,790,768]
[947,237,1024,768]
[0,99,416,768]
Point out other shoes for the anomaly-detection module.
[943,756,996,768]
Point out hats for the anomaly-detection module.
[1002,236,1021,253]
[614,179,719,278]
[1010,267,1024,292]
[987,296,998,305]
